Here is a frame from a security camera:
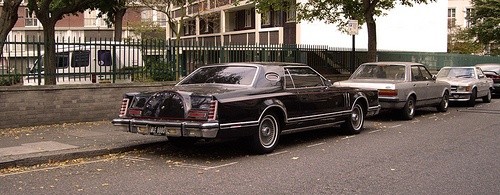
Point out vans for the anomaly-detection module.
[23,40,146,82]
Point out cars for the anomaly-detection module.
[475,64,500,97]
[334,61,452,120]
[436,66,495,106]
[111,61,381,155]
